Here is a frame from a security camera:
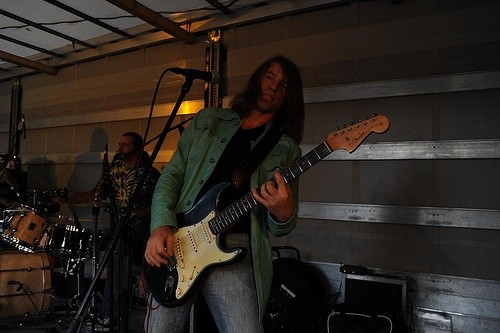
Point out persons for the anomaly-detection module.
[58,132,162,328]
[144,55,305,333]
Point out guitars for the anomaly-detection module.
[143,111,390,309]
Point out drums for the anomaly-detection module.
[0,204,47,252]
[43,222,91,258]
[0,248,52,325]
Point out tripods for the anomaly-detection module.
[54,207,121,333]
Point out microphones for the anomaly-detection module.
[172,67,220,84]
[22,114,27,139]
